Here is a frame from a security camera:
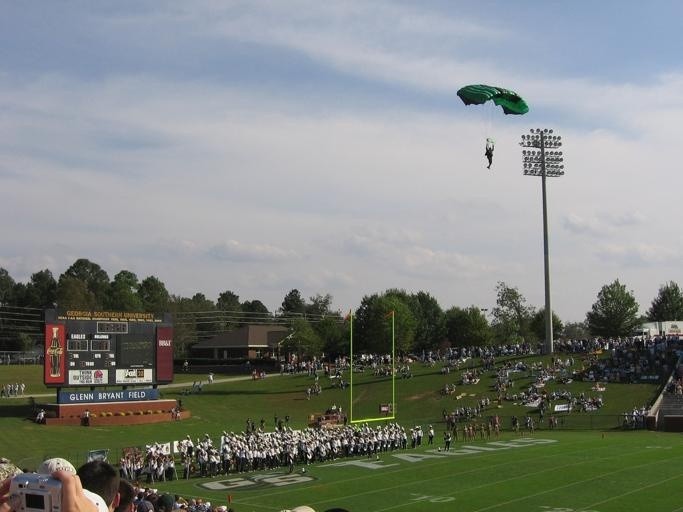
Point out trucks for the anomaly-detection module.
[631,321,683,348]
[306,412,348,429]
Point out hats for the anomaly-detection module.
[38,457,77,477]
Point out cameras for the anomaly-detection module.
[9,473,63,512]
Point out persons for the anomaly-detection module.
[0,333,683,512]
[485,137,495,168]
